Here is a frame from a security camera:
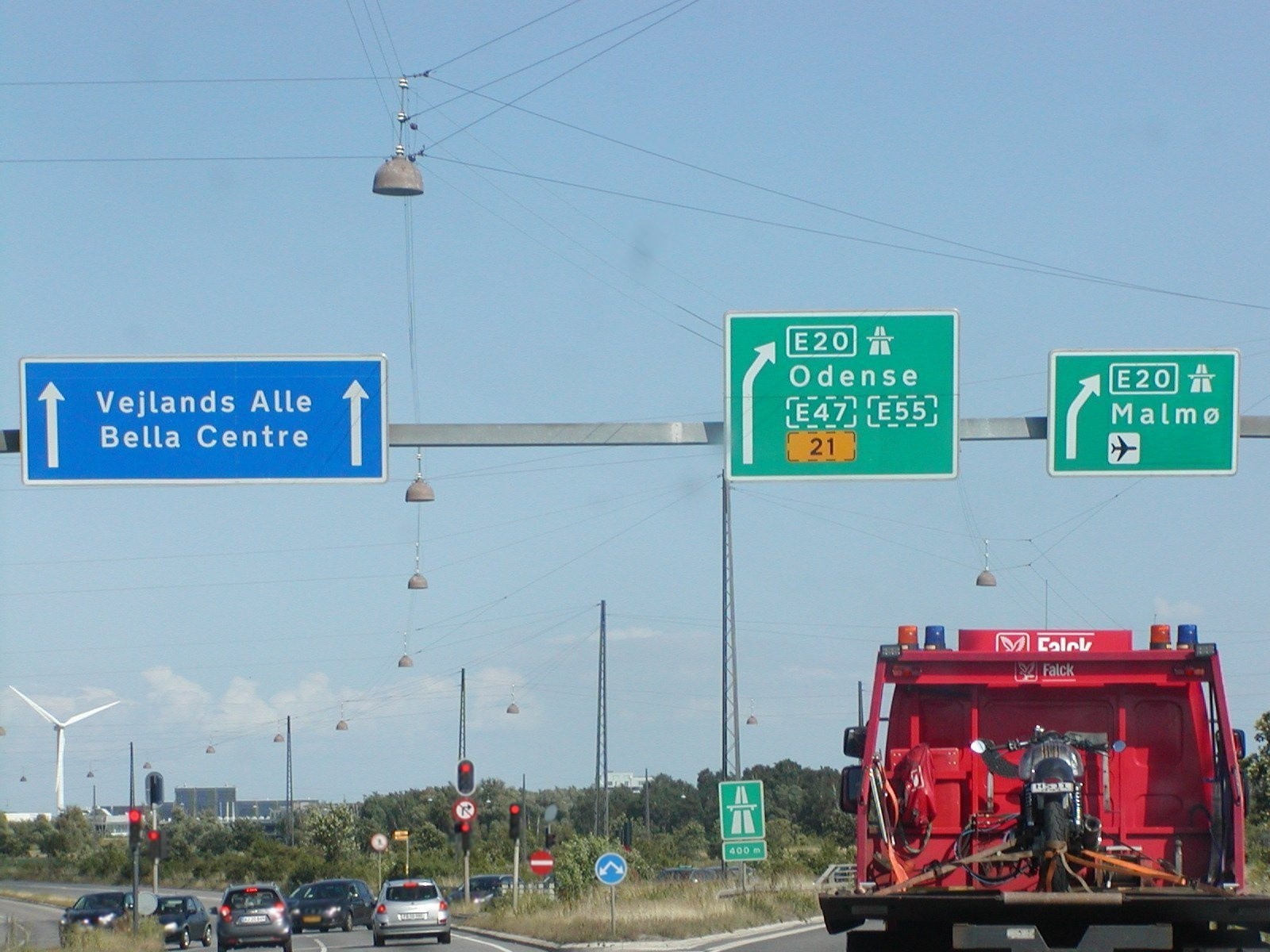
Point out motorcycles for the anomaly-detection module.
[971,722,1128,893]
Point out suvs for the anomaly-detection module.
[370,878,451,947]
[208,881,293,952]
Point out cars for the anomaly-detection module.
[57,890,128,948]
[445,873,523,905]
[283,878,377,934]
[152,894,212,950]
[654,865,754,883]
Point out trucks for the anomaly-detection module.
[816,626,1270,952]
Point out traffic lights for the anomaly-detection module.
[148,830,161,858]
[508,804,519,839]
[546,827,556,848]
[456,759,476,797]
[128,809,140,842]
[460,822,471,850]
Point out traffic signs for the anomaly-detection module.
[17,355,388,484]
[1047,348,1241,477]
[721,308,960,481]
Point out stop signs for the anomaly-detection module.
[530,852,554,874]
